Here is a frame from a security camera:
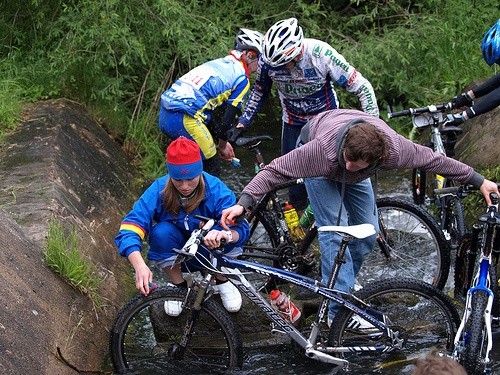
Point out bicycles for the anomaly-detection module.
[432,183,500,375]
[109,216,462,375]
[387,102,468,285]
[236,136,451,305]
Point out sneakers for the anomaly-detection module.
[164,282,189,317]
[216,281,243,313]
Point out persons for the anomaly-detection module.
[158,26,264,179]
[226,17,380,219]
[481,18,500,67]
[220,109,500,335]
[408,347,469,375]
[113,135,251,316]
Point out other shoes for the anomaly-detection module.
[328,313,384,338]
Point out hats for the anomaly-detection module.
[165,135,203,180]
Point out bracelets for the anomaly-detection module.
[221,229,234,244]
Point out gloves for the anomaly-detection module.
[443,108,475,128]
[445,92,473,110]
[226,122,244,143]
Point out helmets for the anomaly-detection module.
[480,18,500,67]
[260,17,304,71]
[233,28,264,58]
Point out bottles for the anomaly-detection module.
[284,201,302,242]
[270,290,301,323]
[436,172,444,189]
[299,204,316,230]
